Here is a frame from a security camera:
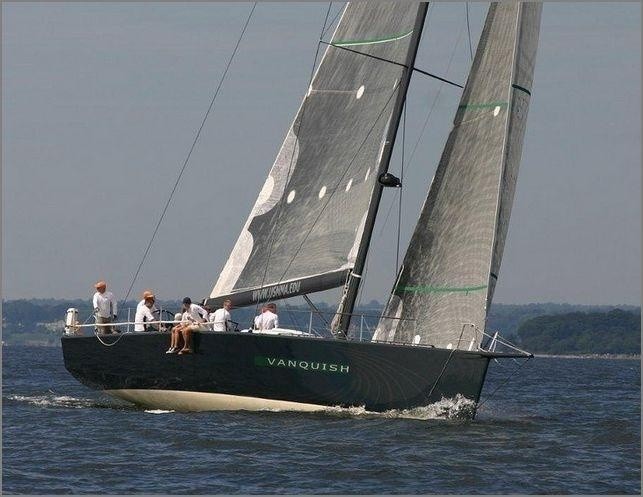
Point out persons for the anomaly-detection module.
[92,281,117,335]
[253,303,279,330]
[135,290,232,356]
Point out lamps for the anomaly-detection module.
[377,172,402,188]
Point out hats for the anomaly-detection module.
[144,292,153,298]
[94,282,106,288]
[183,297,192,304]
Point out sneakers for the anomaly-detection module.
[165,346,192,355]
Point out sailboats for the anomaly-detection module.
[60,1,544,422]
